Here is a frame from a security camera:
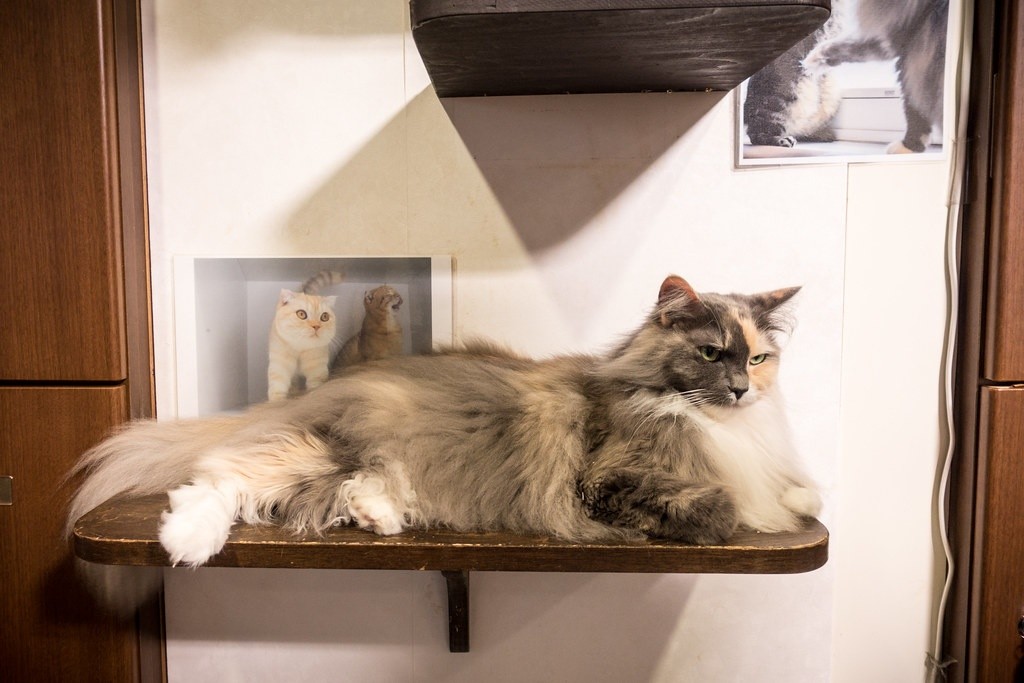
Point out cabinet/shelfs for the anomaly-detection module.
[946,0,1023,682]
[0,0,167,683]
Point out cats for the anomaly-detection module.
[56,274,825,572]
[267,268,404,402]
